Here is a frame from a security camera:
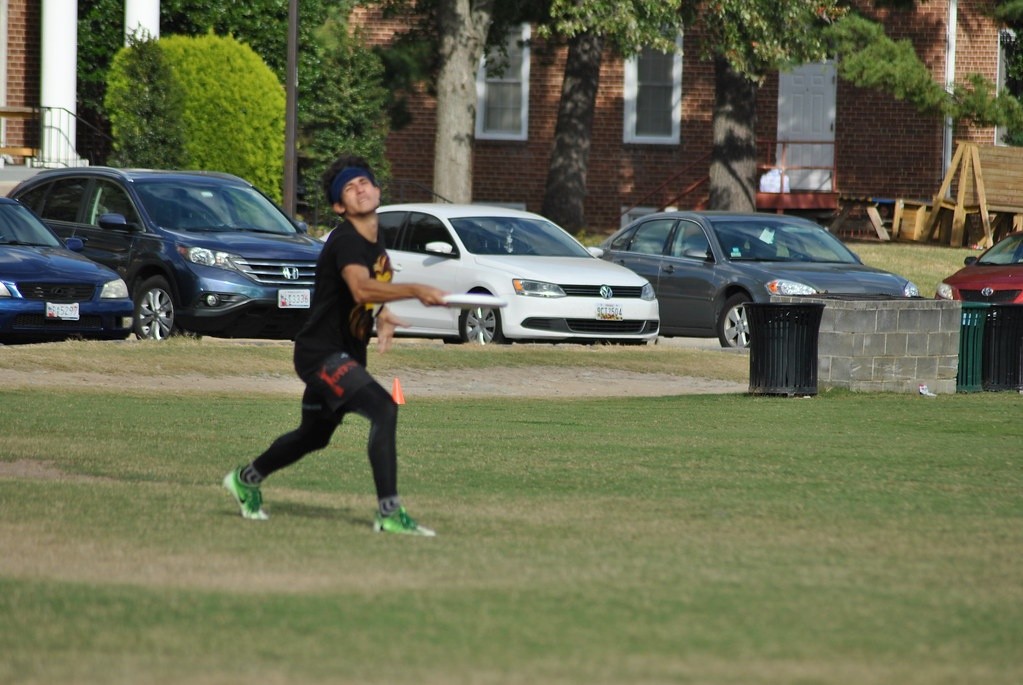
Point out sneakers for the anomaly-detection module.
[222,465,268,519]
[374,506,434,537]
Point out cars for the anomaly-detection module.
[0,197,135,342]
[317,204,661,345]
[935,230,1023,330]
[590,211,920,349]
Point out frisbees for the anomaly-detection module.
[440,291,508,310]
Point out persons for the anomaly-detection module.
[219,153,438,539]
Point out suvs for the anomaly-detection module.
[6,166,325,343]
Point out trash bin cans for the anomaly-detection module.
[956,301,1023,394]
[742,301,826,399]
